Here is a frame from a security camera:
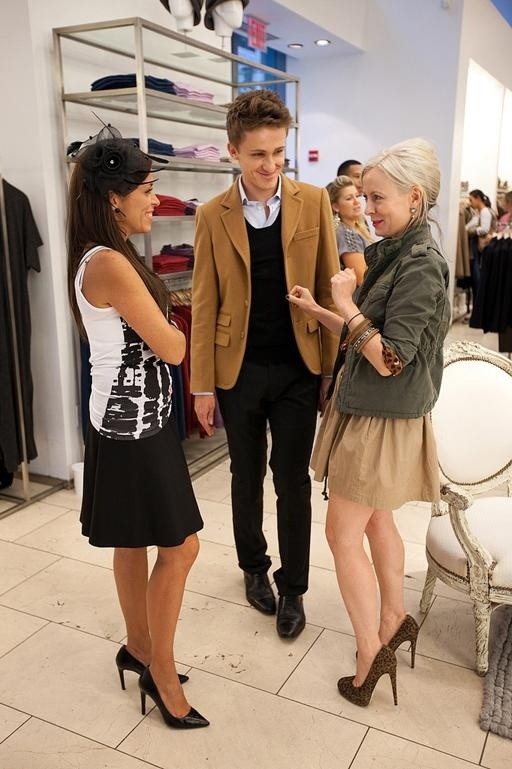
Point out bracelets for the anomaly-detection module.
[344,310,363,325]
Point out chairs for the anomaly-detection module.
[417,335,512,678]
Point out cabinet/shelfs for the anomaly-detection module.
[49,15,307,499]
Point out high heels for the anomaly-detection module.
[337,615,420,707]
[139,665,209,727]
[116,644,189,690]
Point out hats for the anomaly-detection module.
[205,0,249,30]
[162,0,204,25]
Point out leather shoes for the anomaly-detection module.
[276,595,305,638]
[243,570,276,615]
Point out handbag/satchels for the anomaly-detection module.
[479,229,497,251]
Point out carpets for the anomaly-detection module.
[476,602,512,738]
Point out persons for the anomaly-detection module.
[186,88,343,643]
[337,158,365,198]
[483,191,511,244]
[63,131,210,730]
[325,174,376,289]
[463,188,498,250]
[204,0,250,38]
[284,136,452,715]
[161,0,203,31]
